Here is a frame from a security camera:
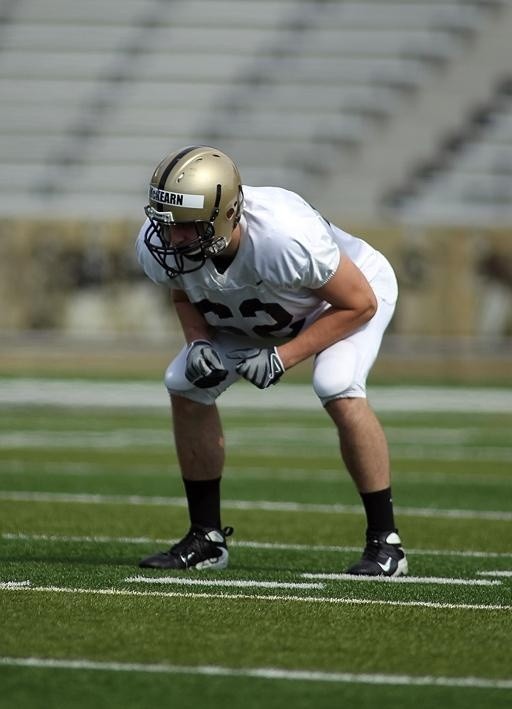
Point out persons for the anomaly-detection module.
[137,147,408,576]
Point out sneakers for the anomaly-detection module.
[137,526,234,571]
[344,528,409,578]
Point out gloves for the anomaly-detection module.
[184,338,229,389]
[226,345,286,390]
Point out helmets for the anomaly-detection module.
[143,144,245,280]
